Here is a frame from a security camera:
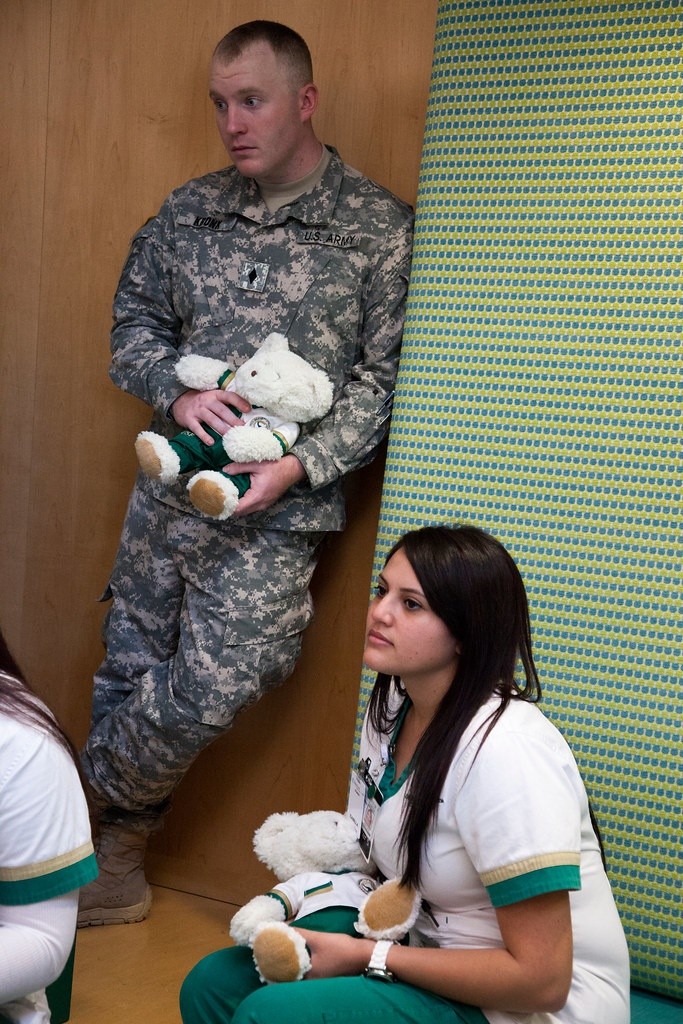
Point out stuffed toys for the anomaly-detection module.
[227,809,426,984]
[133,332,336,521]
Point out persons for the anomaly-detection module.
[0,624,100,1024]
[179,523,632,1024]
[365,809,372,826]
[76,19,416,928]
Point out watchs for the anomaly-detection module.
[364,940,397,985]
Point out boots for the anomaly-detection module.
[77,814,171,928]
